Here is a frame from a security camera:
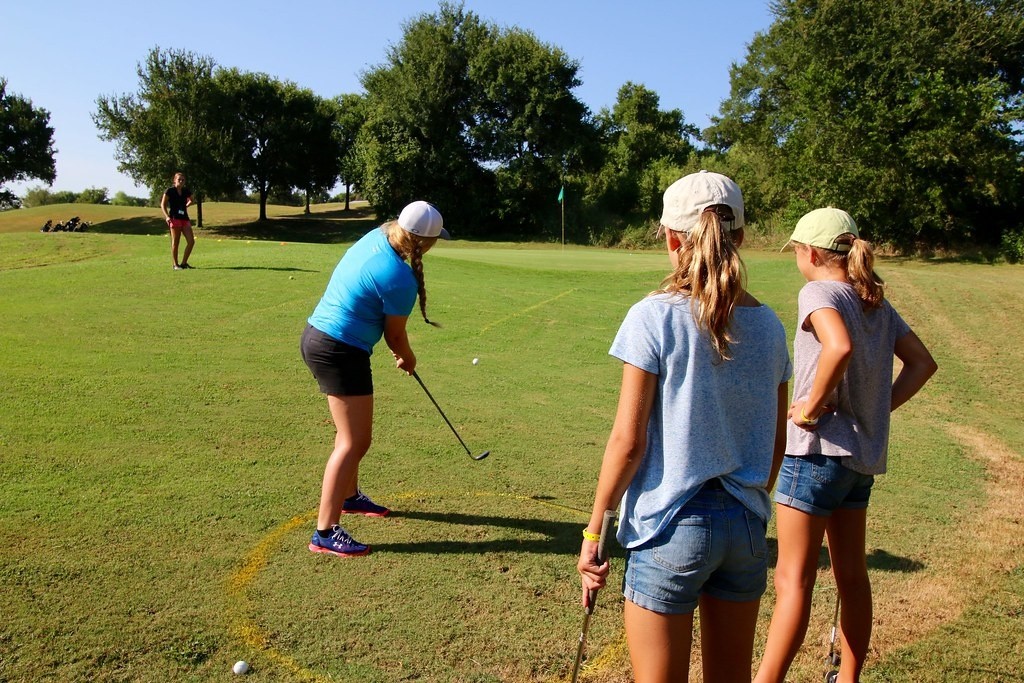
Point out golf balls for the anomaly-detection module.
[234,660,249,675]
[289,276,294,280]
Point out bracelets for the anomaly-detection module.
[802,407,819,425]
[582,528,600,542]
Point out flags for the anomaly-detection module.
[558,188,563,203]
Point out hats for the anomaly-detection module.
[398,201,450,241]
[654,169,744,241]
[781,205,860,253]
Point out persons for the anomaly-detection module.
[752,206,938,683]
[160,173,195,270]
[576,169,794,683]
[299,201,450,557]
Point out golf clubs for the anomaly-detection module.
[169,221,175,271]
[412,370,489,461]
[570,508,618,683]
[827,592,841,666]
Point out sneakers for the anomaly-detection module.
[826,669,840,683]
[341,493,389,518]
[309,528,372,558]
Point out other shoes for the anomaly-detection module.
[180,262,196,269]
[173,264,182,271]
